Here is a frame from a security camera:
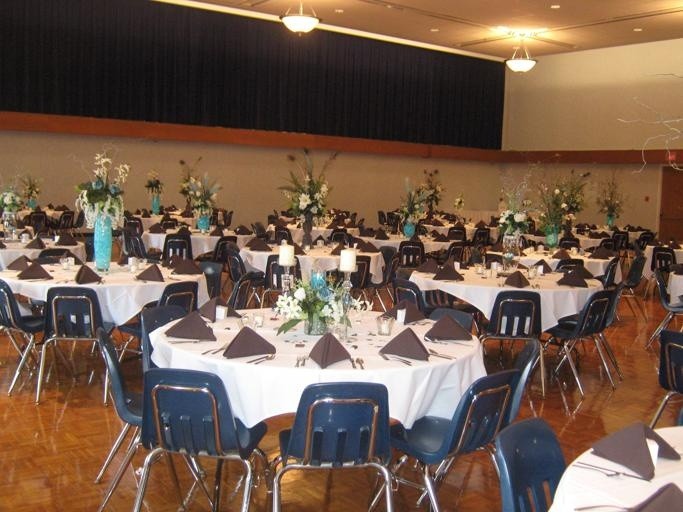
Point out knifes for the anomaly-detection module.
[576,460,649,481]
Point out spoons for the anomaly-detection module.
[428,348,456,359]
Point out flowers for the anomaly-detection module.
[0,172,43,210]
[499,157,622,235]
[180,169,222,218]
[454,193,464,213]
[74,152,128,231]
[145,170,162,196]
[274,150,336,216]
[395,169,441,223]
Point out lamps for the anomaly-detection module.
[280,0,322,37]
[504,31,536,74]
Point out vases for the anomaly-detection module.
[151,195,160,214]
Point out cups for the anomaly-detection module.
[452,261,543,280]
[0,212,393,369]
